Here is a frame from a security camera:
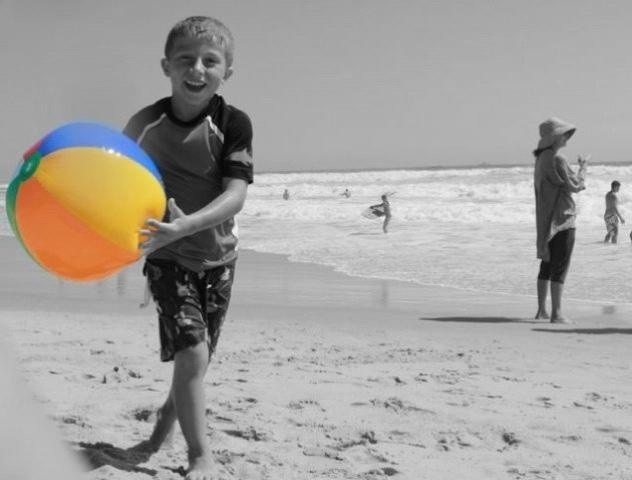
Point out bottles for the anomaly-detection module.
[537,118,577,150]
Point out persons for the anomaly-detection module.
[120,14,254,480]
[532,118,590,323]
[372,195,391,233]
[283,188,289,201]
[343,188,351,198]
[604,181,625,243]
